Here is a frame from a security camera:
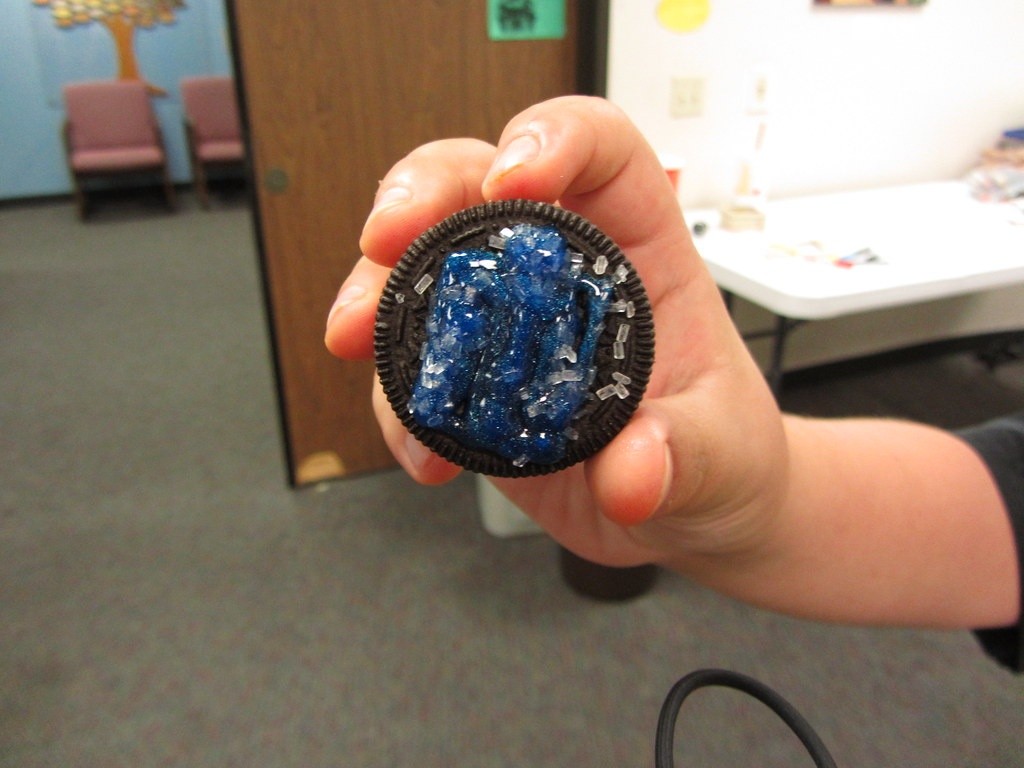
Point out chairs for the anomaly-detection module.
[63,80,178,223]
[182,76,249,212]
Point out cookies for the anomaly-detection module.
[365,195,654,477]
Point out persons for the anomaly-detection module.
[320,93,1024,683]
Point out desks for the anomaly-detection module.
[682,181,1024,412]
